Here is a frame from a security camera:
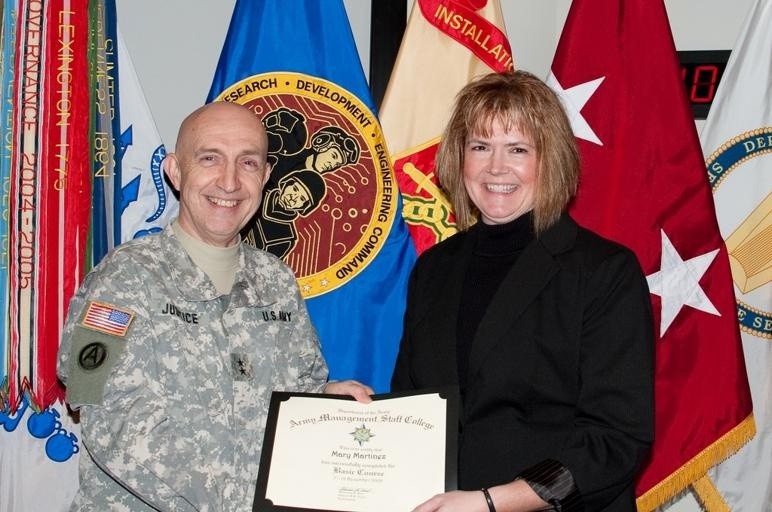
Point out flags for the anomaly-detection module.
[0,0,176,512]
[378,1,515,256]
[545,0,755,512]
[205,1,418,393]
[690,0,772,512]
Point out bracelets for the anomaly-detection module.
[481,486,495,512]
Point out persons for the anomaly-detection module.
[391,74,655,512]
[55,101,375,512]
